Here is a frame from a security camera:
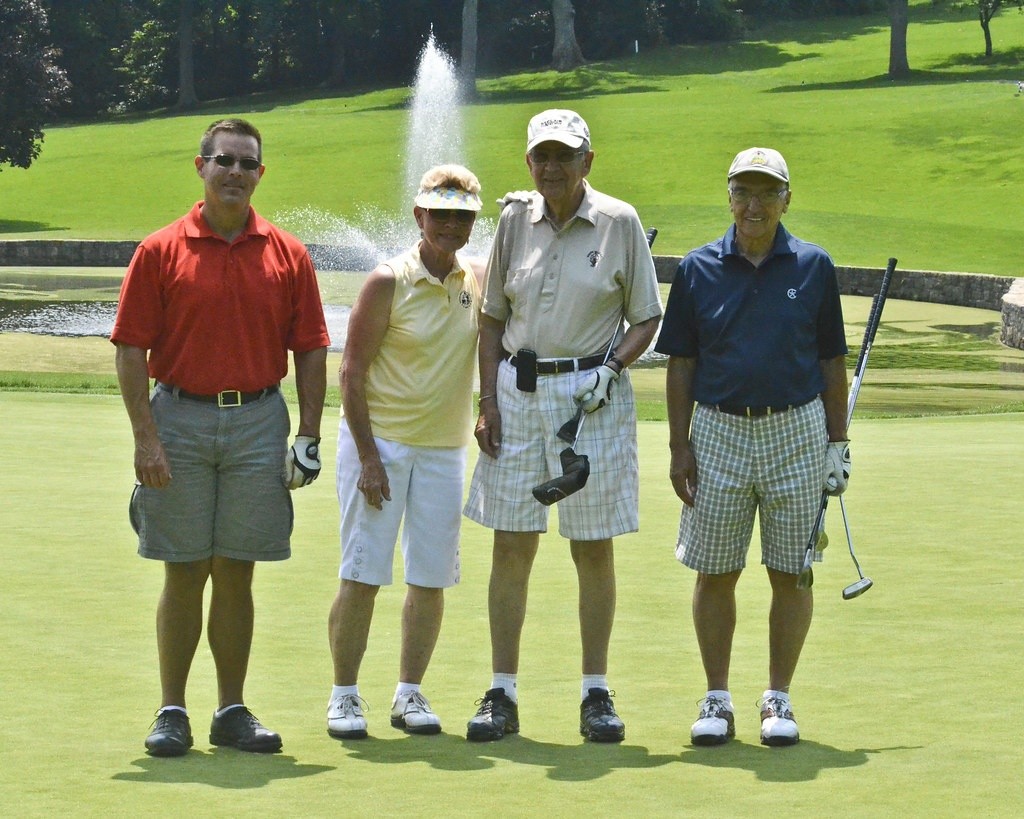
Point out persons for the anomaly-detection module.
[329,163,529,740]
[463,109,662,742]
[111,119,330,756]
[656,146,854,745]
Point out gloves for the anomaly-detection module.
[285,435,321,490]
[823,440,851,496]
[572,365,620,414]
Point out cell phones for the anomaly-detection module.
[517,349,537,392]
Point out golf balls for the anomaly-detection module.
[827,479,839,493]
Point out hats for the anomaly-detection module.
[526,109,590,153]
[415,164,483,211]
[727,146,789,183]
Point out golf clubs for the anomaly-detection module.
[572,228,659,450]
[797,258,899,600]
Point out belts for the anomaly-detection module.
[715,395,817,415]
[158,381,278,407]
[502,348,614,373]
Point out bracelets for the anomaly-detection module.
[610,356,624,373]
[478,392,495,408]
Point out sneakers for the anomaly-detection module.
[326,693,369,737]
[467,687,519,740]
[145,708,193,754]
[391,690,441,733]
[756,686,799,745]
[691,694,735,743]
[579,687,625,740]
[209,706,282,751]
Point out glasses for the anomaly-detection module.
[203,154,260,170]
[728,188,785,204]
[531,152,584,162]
[425,209,477,224]
